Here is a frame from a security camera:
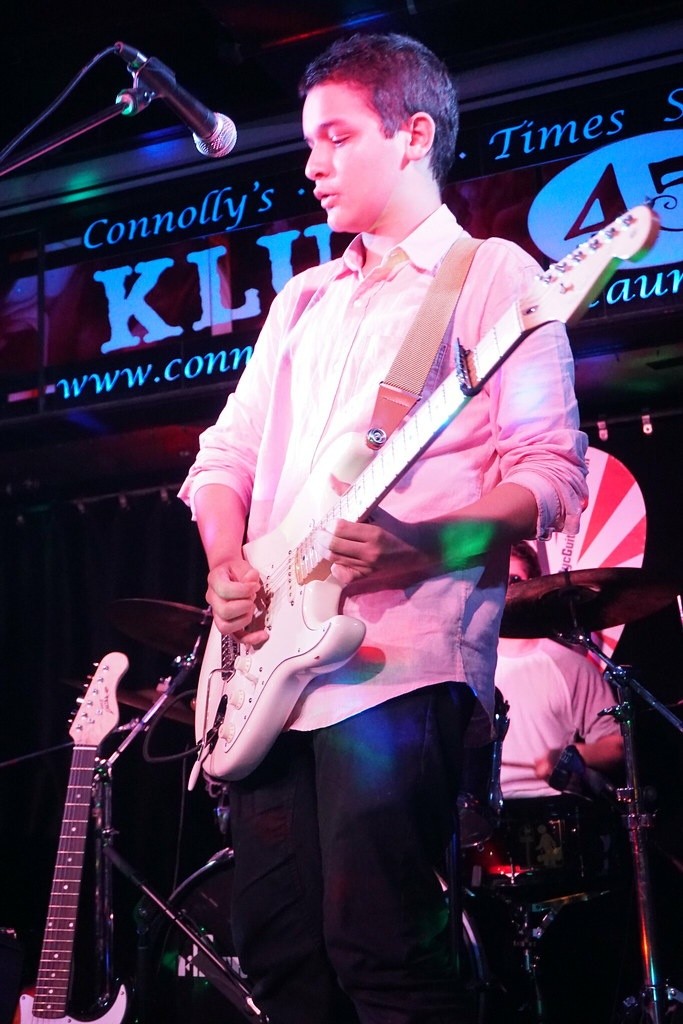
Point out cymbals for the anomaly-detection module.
[499,566,681,639]
[105,597,216,667]
[61,678,198,728]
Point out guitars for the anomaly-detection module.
[10,650,128,1024]
[190,206,662,782]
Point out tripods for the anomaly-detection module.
[559,592,683,1024]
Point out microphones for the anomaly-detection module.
[112,42,237,157]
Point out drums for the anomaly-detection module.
[173,844,491,1023]
[461,794,625,914]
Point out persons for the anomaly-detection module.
[176,34,588,1024]
[494,542,623,798]
